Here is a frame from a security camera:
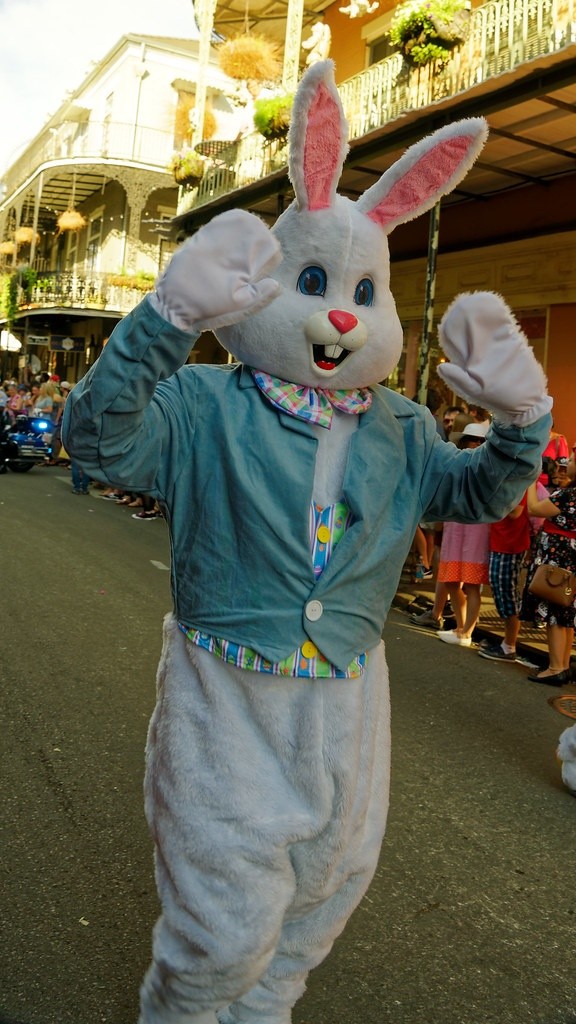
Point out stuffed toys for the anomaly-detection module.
[60,57,553,1024]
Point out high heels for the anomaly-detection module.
[527,669,572,686]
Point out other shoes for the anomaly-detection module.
[72,489,80,495]
[437,630,472,647]
[82,490,90,494]
[38,456,71,470]
[130,511,156,520]
[93,481,143,507]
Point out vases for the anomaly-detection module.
[261,105,293,139]
[173,160,204,185]
[400,8,469,67]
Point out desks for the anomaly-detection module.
[194,140,240,191]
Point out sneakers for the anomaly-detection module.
[477,647,516,663]
[410,610,444,629]
[420,565,434,579]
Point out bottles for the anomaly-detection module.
[415,556,423,583]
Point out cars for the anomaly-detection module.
[0,413,54,473]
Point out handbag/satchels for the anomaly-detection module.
[531,564,576,606]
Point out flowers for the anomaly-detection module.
[252,83,298,136]
[384,0,471,64]
[169,146,209,171]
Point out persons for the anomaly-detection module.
[411,392,576,686]
[62,447,162,520]
[0,372,77,470]
[302,22,325,67]
[245,80,273,130]
[339,0,379,18]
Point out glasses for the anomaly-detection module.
[443,418,454,424]
[471,436,486,444]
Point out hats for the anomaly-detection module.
[56,381,70,389]
[449,423,489,444]
[50,375,59,381]
